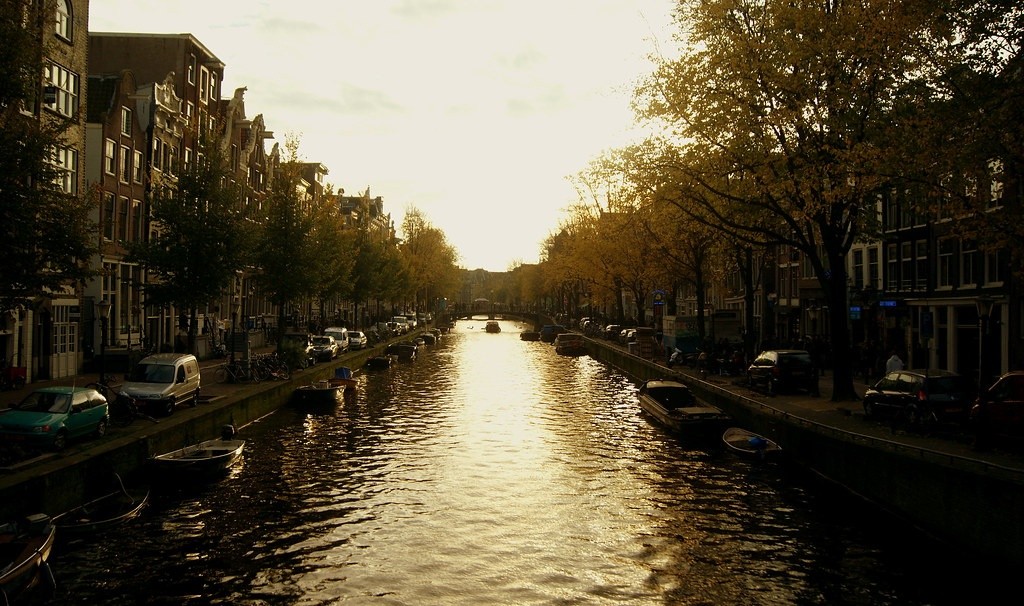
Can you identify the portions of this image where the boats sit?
[486,321,502,333]
[0,514,55,606]
[50,486,150,536]
[151,424,245,473]
[293,366,360,409]
[520,325,584,354]
[637,378,732,435]
[723,428,782,464]
[365,323,455,367]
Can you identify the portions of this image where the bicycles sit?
[213,352,289,383]
[84,375,138,425]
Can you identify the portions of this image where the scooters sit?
[667,341,741,378]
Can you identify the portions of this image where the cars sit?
[579,317,637,343]
[285,311,435,365]
[0,387,110,452]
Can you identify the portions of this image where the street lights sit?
[337,302,342,325]
[491,290,493,300]
[972,291,996,393]
[806,304,821,371]
[230,301,240,365]
[96,298,113,347]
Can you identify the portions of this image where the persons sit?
[886,350,903,385]
[735,329,833,375]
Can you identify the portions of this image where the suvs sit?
[748,349,815,397]
[971,371,1024,441]
[119,353,201,417]
[863,369,968,439]
